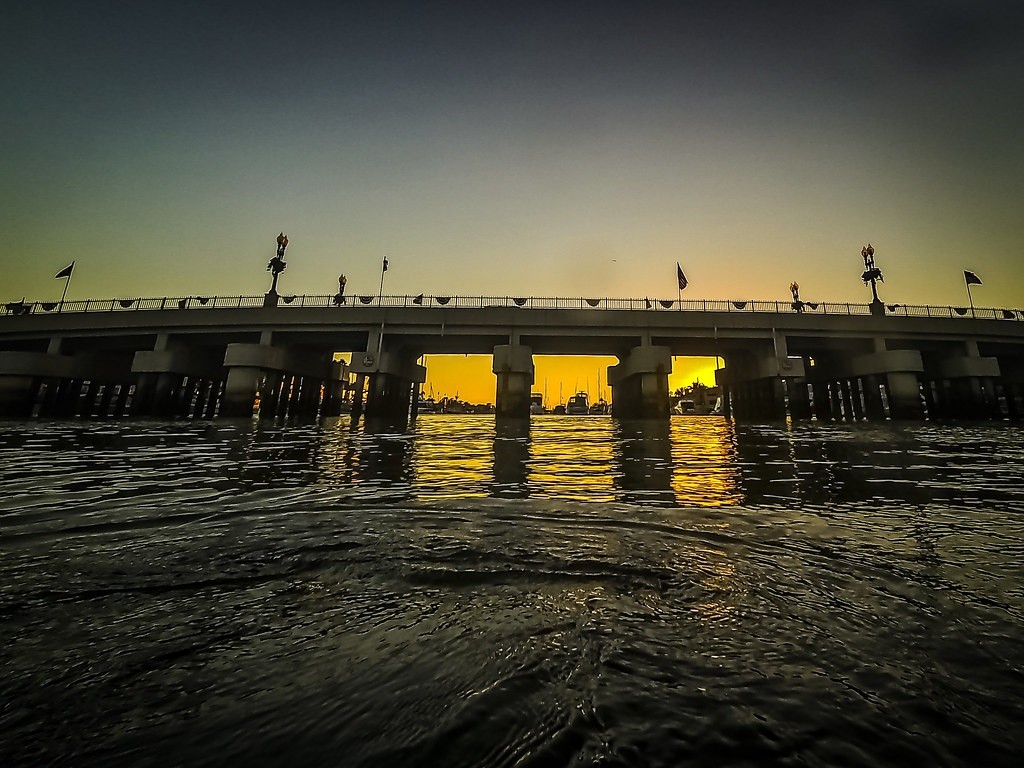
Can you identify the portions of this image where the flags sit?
[963,270,984,285]
[54,264,73,277]
[679,269,688,291]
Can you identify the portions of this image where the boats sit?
[335,389,734,418]
